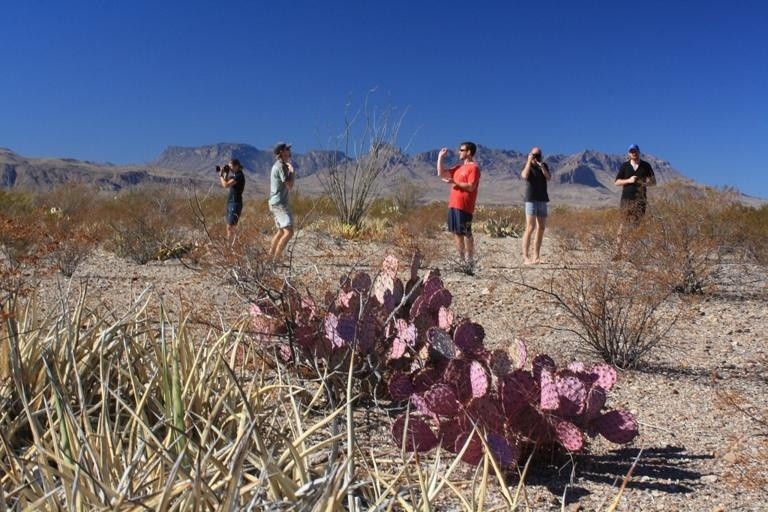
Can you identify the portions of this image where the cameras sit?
[216,165,229,174]
[533,153,542,162]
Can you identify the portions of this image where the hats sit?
[272,141,293,156]
[626,143,640,153]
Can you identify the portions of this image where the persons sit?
[216,158,245,242]
[609,142,657,262]
[266,142,297,269]
[436,141,481,269]
[519,147,551,266]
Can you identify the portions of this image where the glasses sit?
[459,148,470,152]
